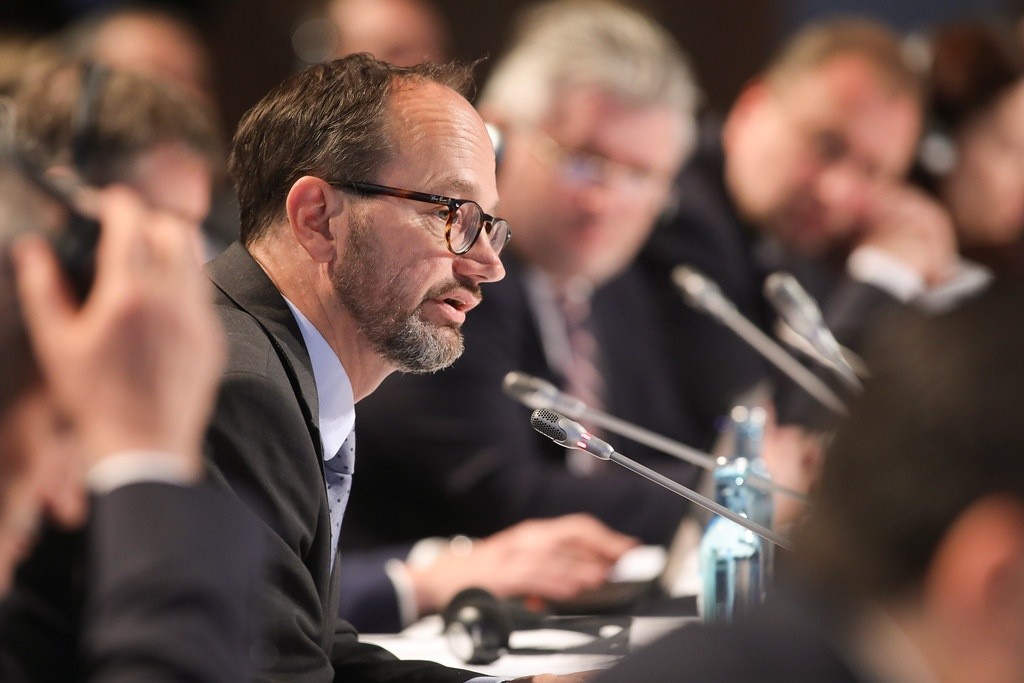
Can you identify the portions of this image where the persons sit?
[1,0,1024,683]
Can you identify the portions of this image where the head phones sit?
[440,587,634,665]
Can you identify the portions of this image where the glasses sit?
[508,122,671,205]
[286,181,512,256]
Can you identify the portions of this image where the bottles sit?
[697,464,765,624]
[733,420,776,531]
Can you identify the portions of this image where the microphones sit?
[500,262,872,550]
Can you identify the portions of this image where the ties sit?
[556,278,600,474]
[318,430,353,576]
